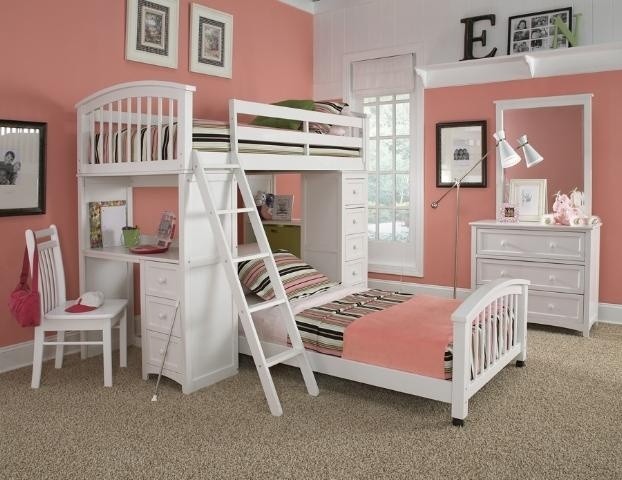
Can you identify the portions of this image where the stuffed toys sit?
[552,191,573,226]
[570,187,583,214]
[254,191,273,221]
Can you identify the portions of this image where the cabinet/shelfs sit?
[300,170,370,292]
[140,259,242,395]
[468,218,603,337]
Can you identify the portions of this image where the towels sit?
[342,292,508,380]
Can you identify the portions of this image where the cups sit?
[121,226,141,249]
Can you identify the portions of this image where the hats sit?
[65,290,105,314]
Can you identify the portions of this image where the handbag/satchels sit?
[7,282,41,328]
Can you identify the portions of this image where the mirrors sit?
[492,94,593,223]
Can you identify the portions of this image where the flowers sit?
[567,186,584,213]
[552,190,577,225]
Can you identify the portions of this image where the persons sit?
[0,151,21,185]
[513,14,566,53]
[454,149,469,160]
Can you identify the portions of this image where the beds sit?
[72,81,370,394]
[226,241,531,425]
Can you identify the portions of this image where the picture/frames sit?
[0,118,48,217]
[272,194,294,221]
[125,0,180,69]
[507,7,572,56]
[189,2,233,79]
[509,178,546,221]
[435,119,486,188]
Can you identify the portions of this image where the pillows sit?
[250,98,315,130]
[234,248,342,304]
[301,98,349,134]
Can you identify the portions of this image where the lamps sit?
[430,130,523,299]
[512,134,544,170]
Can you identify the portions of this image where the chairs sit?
[25,224,130,388]
